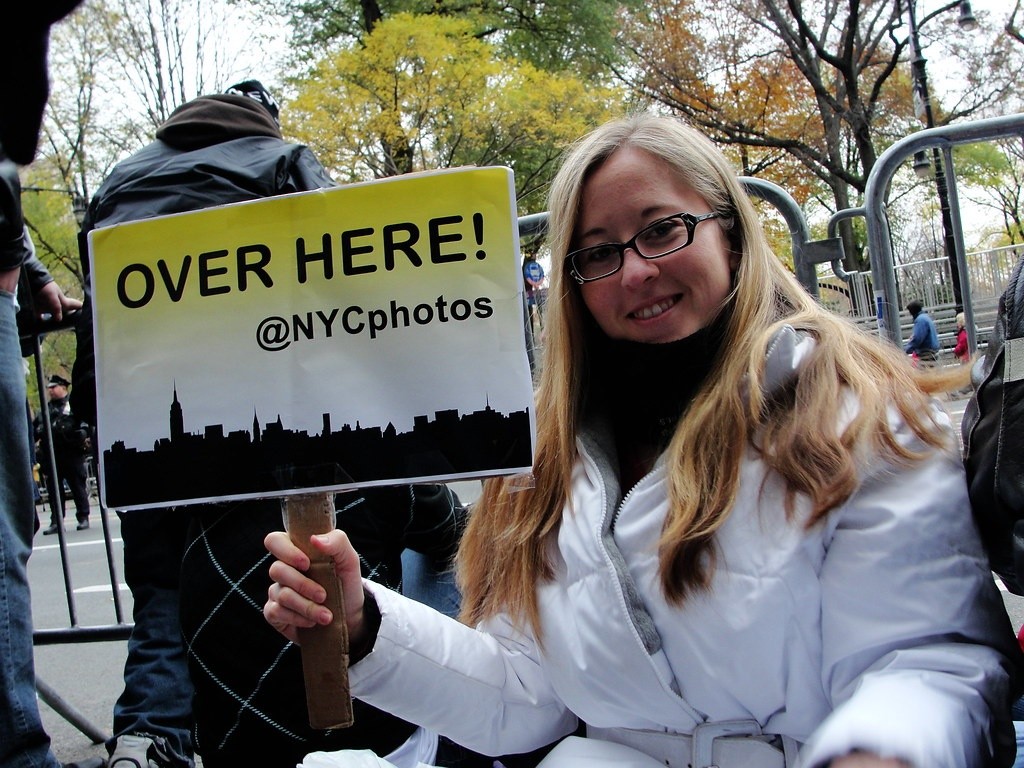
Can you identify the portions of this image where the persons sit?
[904,300,959,402]
[264,116,1024,768]
[954,312,973,397]
[0,0,107,768]
[31,375,90,535]
[69,79,472,768]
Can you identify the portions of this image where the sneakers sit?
[102,731,184,768]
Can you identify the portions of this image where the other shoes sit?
[41,524,57,537]
[74,518,92,532]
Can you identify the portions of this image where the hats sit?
[222,76,281,125]
[906,301,922,315]
[43,374,72,389]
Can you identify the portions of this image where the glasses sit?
[562,208,732,285]
